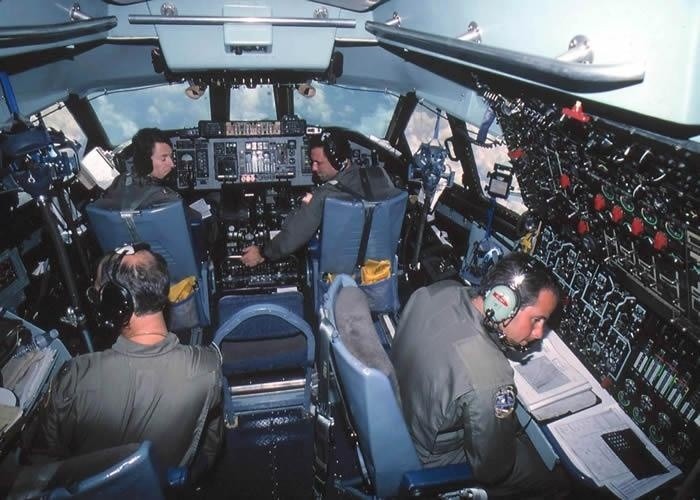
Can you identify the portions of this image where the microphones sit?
[482,318,540,353]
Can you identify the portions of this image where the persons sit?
[238,127,396,270]
[99,126,192,223]
[20,239,225,482]
[387,251,575,500]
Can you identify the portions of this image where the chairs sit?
[84,198,216,347]
[207,291,320,447]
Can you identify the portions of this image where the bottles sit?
[11,328,60,358]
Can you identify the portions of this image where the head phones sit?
[133,128,160,177]
[483,259,539,323]
[320,130,348,172]
[92,241,151,338]
